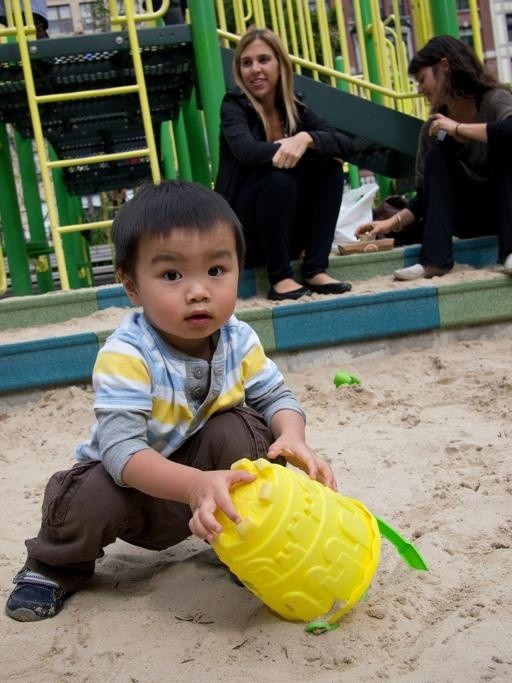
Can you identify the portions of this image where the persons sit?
[2,178,340,623]
[212,27,355,301]
[352,34,512,281]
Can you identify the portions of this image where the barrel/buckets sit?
[209,458,428,634]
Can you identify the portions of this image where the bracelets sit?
[455,122,463,136]
[393,214,402,232]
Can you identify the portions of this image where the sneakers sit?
[302,279,353,295]
[4,567,77,622]
[267,284,314,302]
[393,262,447,281]
